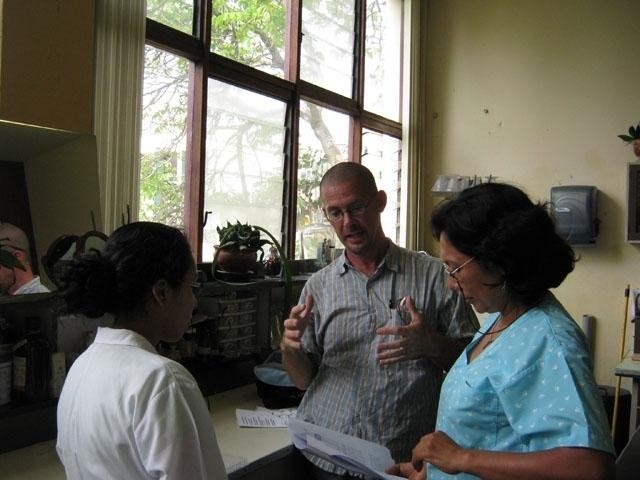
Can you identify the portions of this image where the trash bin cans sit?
[597,385,631,458]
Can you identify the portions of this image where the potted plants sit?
[617,123,640,156]
[213,220,272,273]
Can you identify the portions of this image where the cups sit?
[431,174,495,192]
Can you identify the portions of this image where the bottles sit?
[264,247,282,277]
[0,312,53,406]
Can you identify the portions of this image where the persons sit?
[0,223,51,295]
[386,181,617,480]
[54,221,229,480]
[279,162,481,480]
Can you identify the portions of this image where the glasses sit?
[327,194,378,223]
[442,255,478,281]
